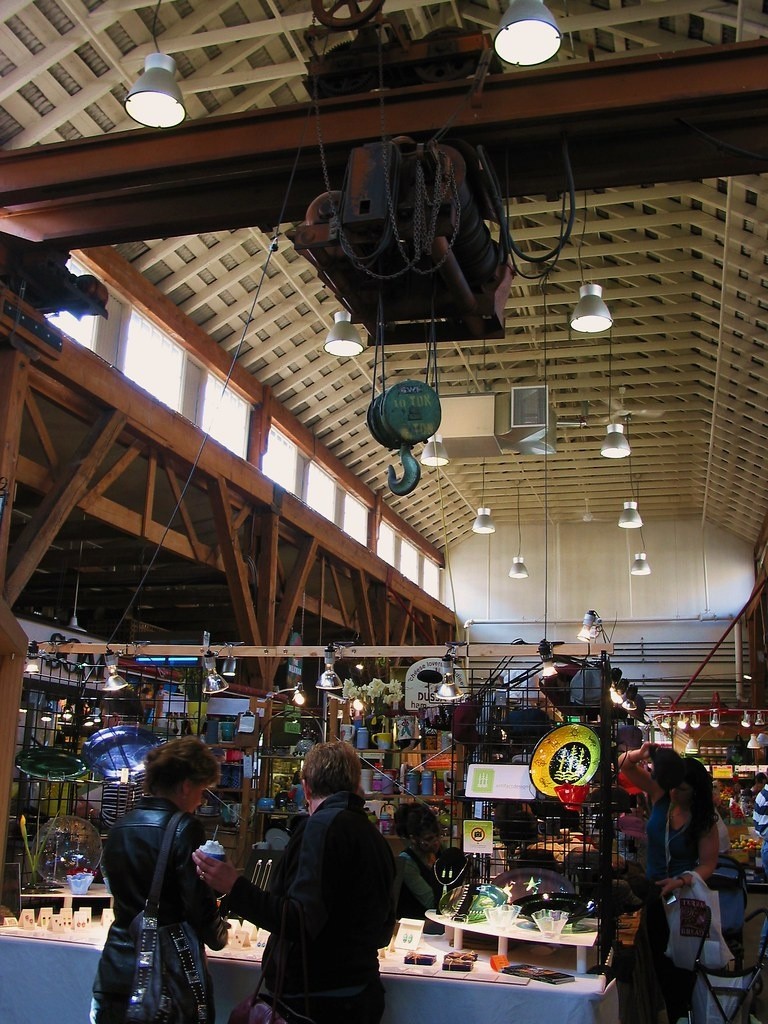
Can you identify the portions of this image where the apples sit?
[731,839,762,849]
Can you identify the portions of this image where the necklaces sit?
[667,810,681,821]
[410,846,436,868]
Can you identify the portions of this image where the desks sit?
[20,883,114,915]
[0,909,606,1024]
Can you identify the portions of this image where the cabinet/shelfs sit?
[685,738,755,780]
[140,694,464,878]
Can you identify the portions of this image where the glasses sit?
[415,832,447,845]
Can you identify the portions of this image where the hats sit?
[612,879,642,909]
[452,703,479,745]
[647,744,683,790]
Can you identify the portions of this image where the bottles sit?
[356,727,451,833]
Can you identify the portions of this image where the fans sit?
[566,498,614,523]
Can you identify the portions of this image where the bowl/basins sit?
[395,739,421,751]
[201,806,214,814]
[510,892,598,926]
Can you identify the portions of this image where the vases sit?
[365,715,382,749]
[188,702,208,735]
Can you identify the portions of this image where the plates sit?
[439,884,508,922]
[198,813,220,816]
[265,828,290,850]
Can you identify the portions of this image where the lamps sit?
[222,656,236,676]
[124,0,186,129]
[421,435,449,467]
[83,699,101,726]
[747,734,760,749]
[67,513,88,632]
[508,485,529,578]
[103,649,128,690]
[741,714,750,728]
[568,190,613,332]
[471,457,495,533]
[661,715,671,728]
[62,705,72,718]
[619,416,644,528]
[315,643,343,689]
[755,713,765,726]
[631,479,650,575]
[324,311,365,356]
[538,639,558,677]
[576,610,596,642]
[41,702,52,722]
[609,667,637,711]
[600,328,633,459]
[436,654,464,699]
[709,711,720,727]
[202,650,229,693]
[678,713,689,730]
[688,714,701,728]
[685,738,698,754]
[494,0,562,66]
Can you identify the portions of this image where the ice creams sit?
[198,839,225,854]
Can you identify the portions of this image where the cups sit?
[199,850,226,863]
[251,842,271,850]
[372,733,392,750]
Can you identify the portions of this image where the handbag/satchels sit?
[129,922,221,1023]
[664,874,736,969]
[227,991,322,1024]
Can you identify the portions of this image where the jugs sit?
[227,804,242,822]
[339,724,355,746]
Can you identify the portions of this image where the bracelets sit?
[677,877,686,888]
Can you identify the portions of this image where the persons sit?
[89,735,231,1024]
[140,684,164,727]
[168,712,178,737]
[192,741,397,1024]
[181,715,191,738]
[101,712,120,730]
[389,802,448,921]
[619,741,768,1024]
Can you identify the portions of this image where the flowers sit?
[173,667,210,702]
[341,678,405,726]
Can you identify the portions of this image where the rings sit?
[200,871,207,881]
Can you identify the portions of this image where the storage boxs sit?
[405,954,436,966]
[442,962,473,971]
[444,953,478,962]
[727,850,749,862]
